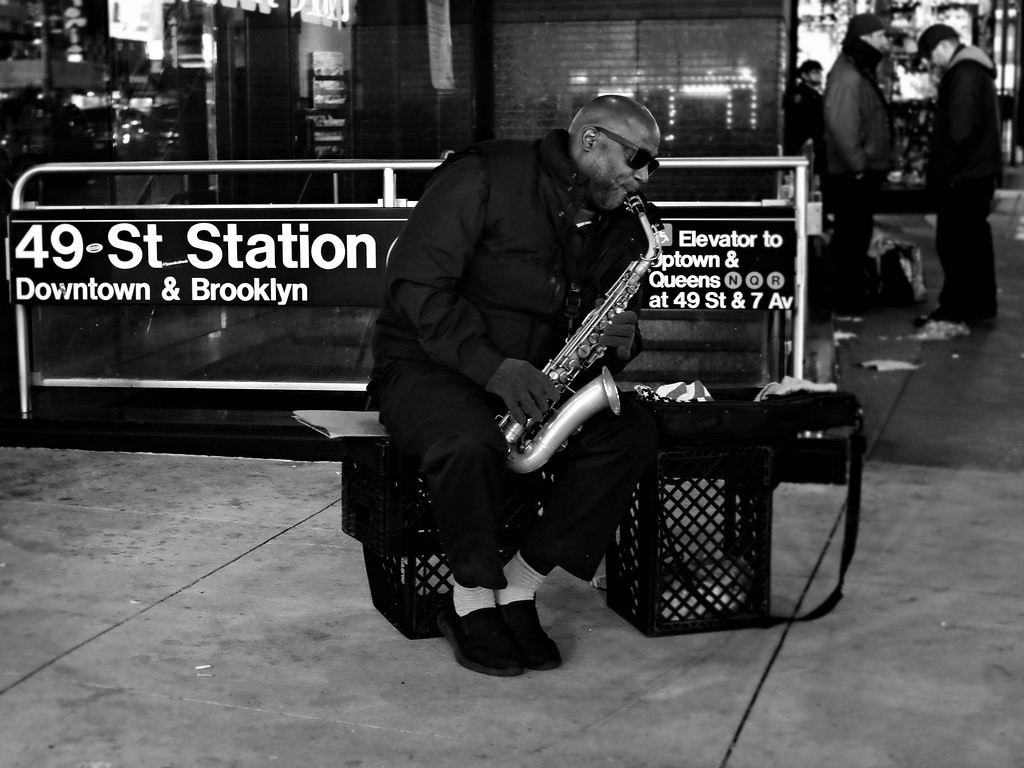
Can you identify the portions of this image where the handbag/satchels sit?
[867,233,929,307]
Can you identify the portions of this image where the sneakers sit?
[437,599,562,676]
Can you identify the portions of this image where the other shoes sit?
[914,315,994,329]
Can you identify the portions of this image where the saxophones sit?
[497,190,686,473]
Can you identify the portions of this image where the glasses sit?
[594,126,660,177]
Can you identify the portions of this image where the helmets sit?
[797,60,823,77]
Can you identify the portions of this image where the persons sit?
[815,12,889,320]
[367,95,663,676]
[910,24,1000,327]
[782,61,833,234]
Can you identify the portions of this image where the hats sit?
[847,13,885,37]
[912,24,960,67]
